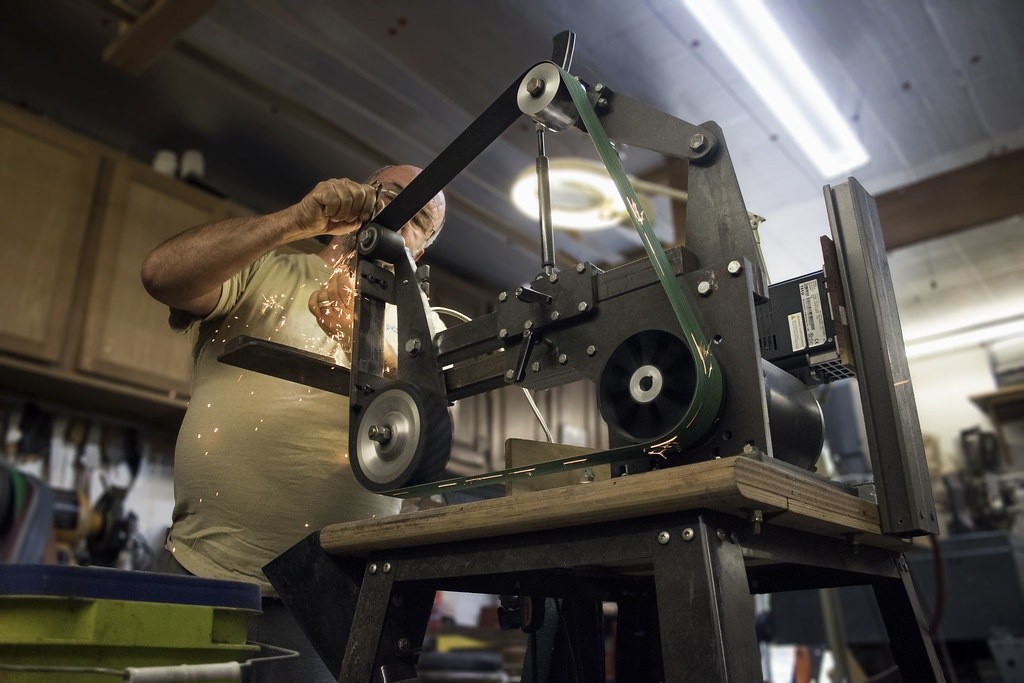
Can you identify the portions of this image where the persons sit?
[140,165,449,683]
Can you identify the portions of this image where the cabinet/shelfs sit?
[0,109,608,479]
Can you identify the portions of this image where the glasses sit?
[375,189,436,242]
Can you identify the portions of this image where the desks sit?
[319,456,944,683]
